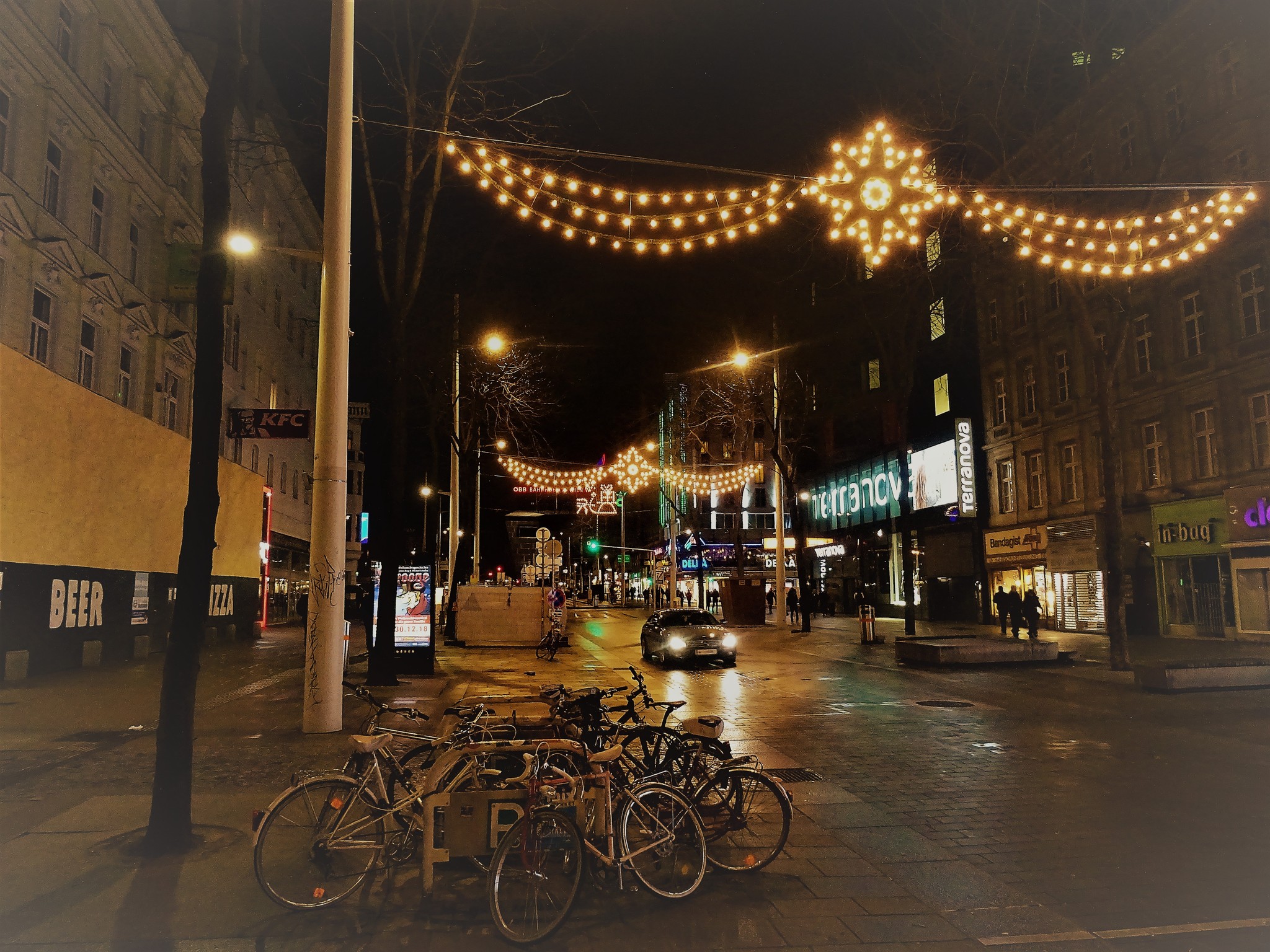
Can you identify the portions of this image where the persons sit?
[854,588,864,617]
[812,589,818,619]
[993,586,1009,634]
[706,589,712,611]
[786,588,799,624]
[1023,590,1043,638]
[819,588,827,617]
[625,586,692,609]
[766,590,776,614]
[711,589,720,613]
[827,587,838,615]
[1007,585,1023,637]
[296,594,308,654]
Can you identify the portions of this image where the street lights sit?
[733,320,791,627]
[420,472,433,553]
[645,442,678,611]
[451,331,505,625]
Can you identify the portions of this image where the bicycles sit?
[249,668,796,944]
[532,616,565,661]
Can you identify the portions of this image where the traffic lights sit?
[497,567,501,572]
[515,579,520,583]
[488,572,493,577]
[589,542,599,551]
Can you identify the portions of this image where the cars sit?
[640,606,738,664]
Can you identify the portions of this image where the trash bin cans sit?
[857,604,876,643]
